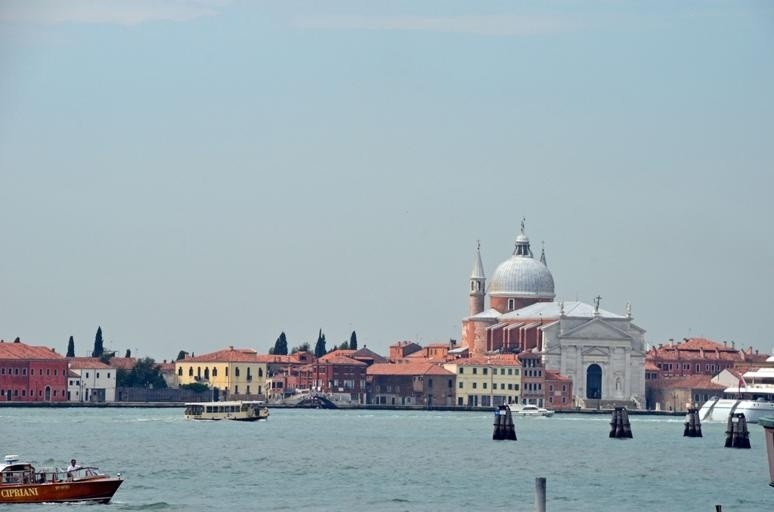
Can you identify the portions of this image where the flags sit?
[739,375,744,384]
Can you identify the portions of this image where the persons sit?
[66,458,82,482]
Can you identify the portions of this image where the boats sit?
[518,404,555,417]
[0,454,125,505]
[183,400,270,420]
[698,356,774,425]
